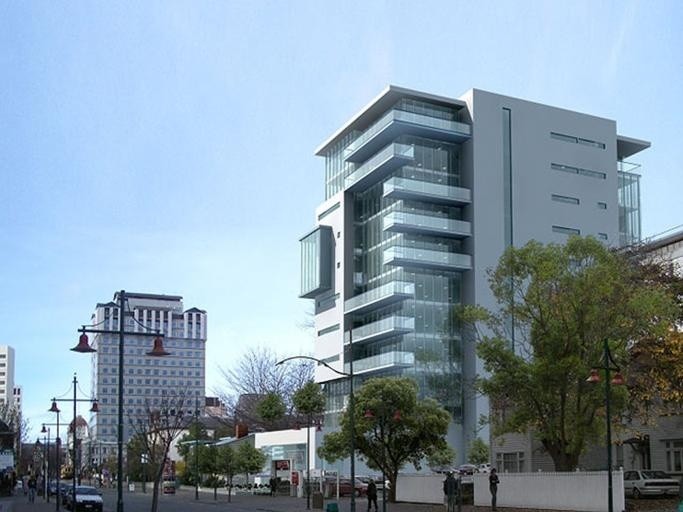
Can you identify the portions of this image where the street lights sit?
[275,316,356,512]
[37,372,100,512]
[364,400,400,512]
[70,290,171,512]
[586,337,626,512]
[296,415,321,510]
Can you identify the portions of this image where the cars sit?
[431,463,491,475]
[326,476,383,497]
[624,469,680,500]
[50,482,103,512]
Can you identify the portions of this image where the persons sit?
[22,477,29,498]
[443,471,457,511]
[27,475,37,504]
[365,478,378,512]
[488,467,499,511]
[269,474,277,496]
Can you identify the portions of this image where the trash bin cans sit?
[312,492,324,509]
